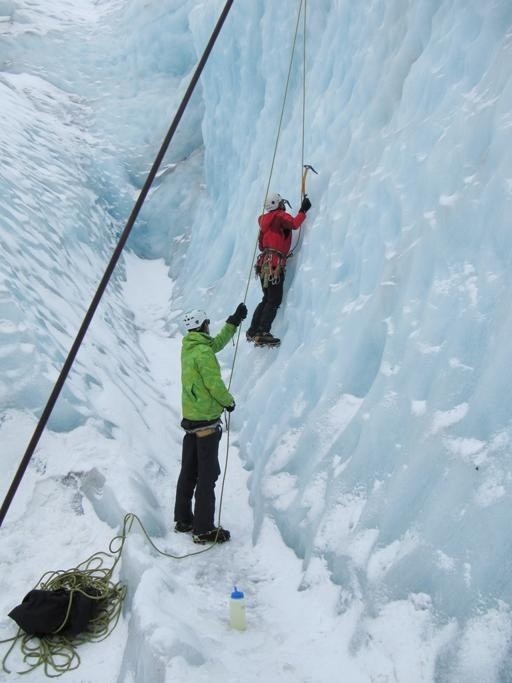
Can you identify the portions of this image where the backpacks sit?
[7,588,91,633]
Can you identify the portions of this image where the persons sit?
[246,195,314,347]
[174,303,244,544]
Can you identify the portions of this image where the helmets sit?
[184,308,210,329]
[264,191,282,212]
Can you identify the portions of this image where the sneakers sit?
[246,331,281,350]
[174,521,230,545]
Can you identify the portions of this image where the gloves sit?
[227,304,247,327]
[299,198,312,214]
[227,401,236,413]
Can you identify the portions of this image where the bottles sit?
[228,584,247,631]
